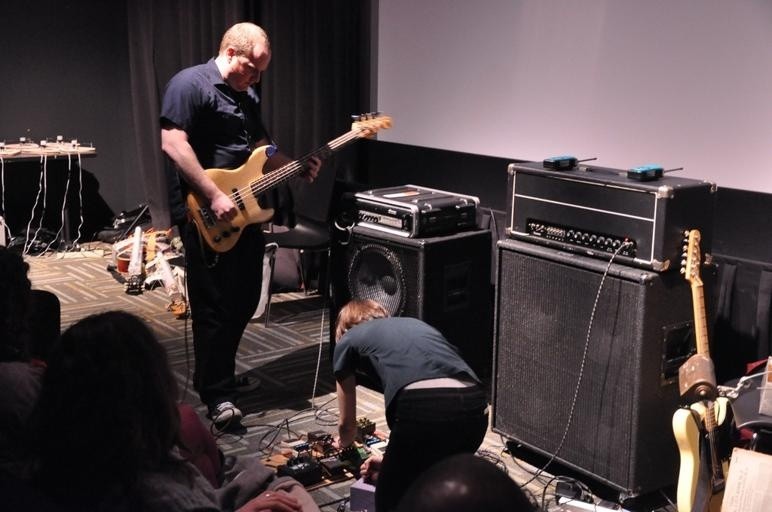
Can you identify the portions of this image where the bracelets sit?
[337,437,355,451]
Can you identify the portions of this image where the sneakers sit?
[212,399,243,426]
[218,375,261,393]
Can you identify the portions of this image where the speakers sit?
[331,226,492,395]
[491,240,718,496]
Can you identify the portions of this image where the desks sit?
[0,142,98,242]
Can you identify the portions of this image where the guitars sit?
[671,230,737,512]
[187,112,393,252]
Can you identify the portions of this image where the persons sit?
[332,297,489,510]
[155,23,321,428]
[1,243,237,491]
[393,453,539,511]
[36,314,324,512]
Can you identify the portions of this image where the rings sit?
[266,493,271,497]
